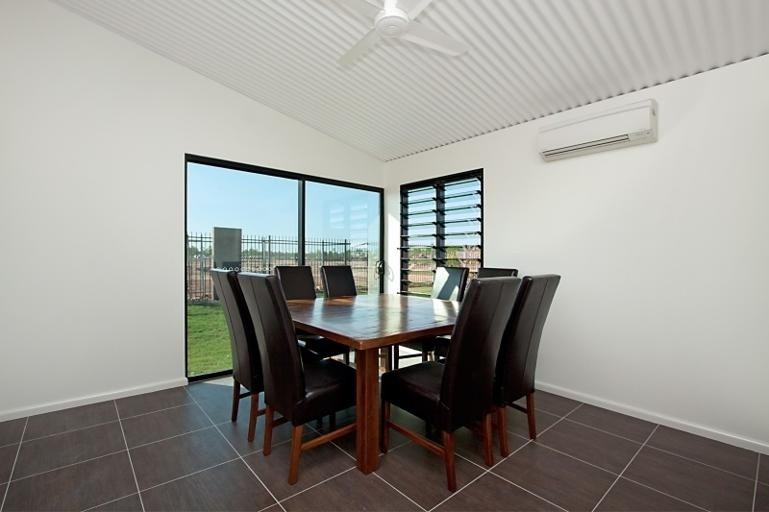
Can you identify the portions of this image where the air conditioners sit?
[539,98,659,163]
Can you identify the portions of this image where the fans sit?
[336,0,469,67]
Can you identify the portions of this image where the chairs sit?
[392,266,467,370]
[427,273,560,458]
[210,268,337,455]
[321,266,358,361]
[379,276,522,491]
[434,267,519,360]
[275,265,349,365]
[238,272,360,485]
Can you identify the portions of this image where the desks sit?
[283,295,461,475]
